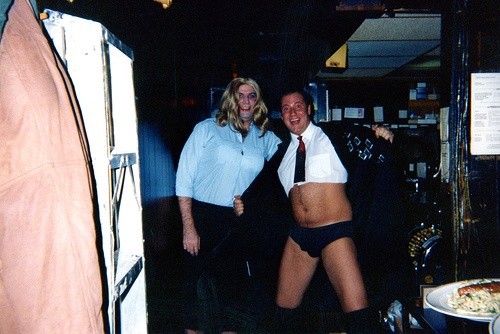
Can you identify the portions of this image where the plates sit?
[489,313,500,334]
[425,278,500,323]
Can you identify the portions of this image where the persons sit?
[175,78,284,333]
[233,87,394,334]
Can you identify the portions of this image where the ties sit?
[293,136,306,183]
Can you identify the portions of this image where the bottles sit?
[406,164,420,204]
